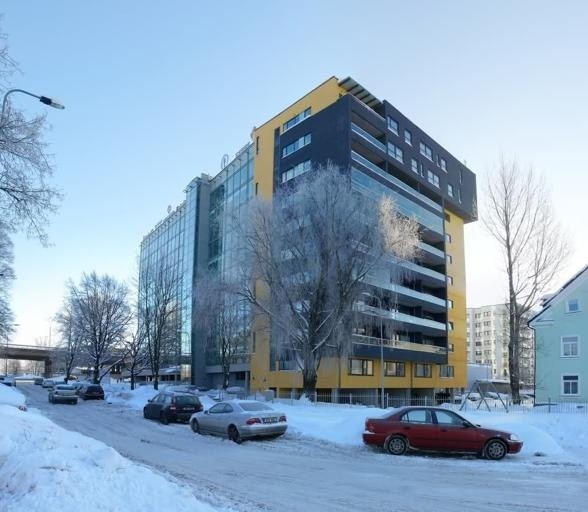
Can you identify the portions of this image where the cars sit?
[0,376,16,387]
[362,406,524,460]
[143,391,289,444]
[34,377,104,405]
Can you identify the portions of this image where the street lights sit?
[5,324,21,376]
[0,89,66,163]
